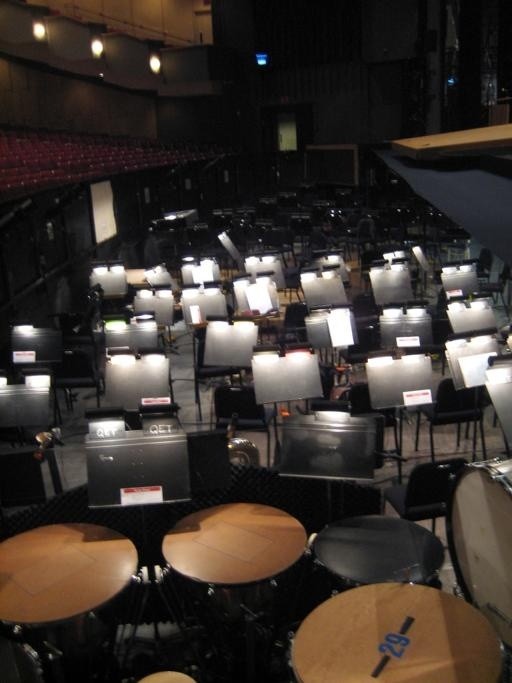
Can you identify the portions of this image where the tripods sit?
[161,325,181,355]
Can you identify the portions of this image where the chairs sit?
[384,458,469,537]
[471,248,510,317]
[191,326,244,421]
[49,336,104,413]
[132,198,432,280]
[209,377,490,462]
[279,302,313,348]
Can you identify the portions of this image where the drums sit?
[445,457,511,648]
[289,579,506,683]
[162,503,309,618]
[1,522,141,644]
[311,515,445,602]
